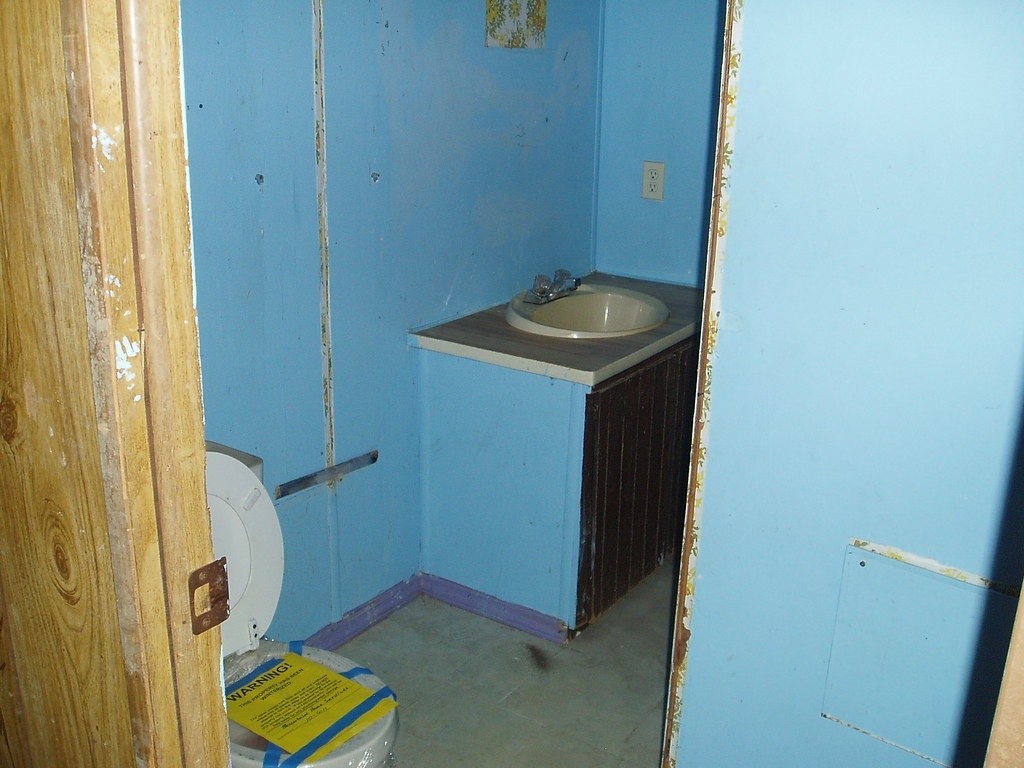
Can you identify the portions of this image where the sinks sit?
[504,280,671,340]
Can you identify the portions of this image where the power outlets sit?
[642,162,663,200]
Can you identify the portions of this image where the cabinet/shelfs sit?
[574,336,700,628]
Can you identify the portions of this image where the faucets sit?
[539,272,583,304]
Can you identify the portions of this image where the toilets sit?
[205,435,401,766]
